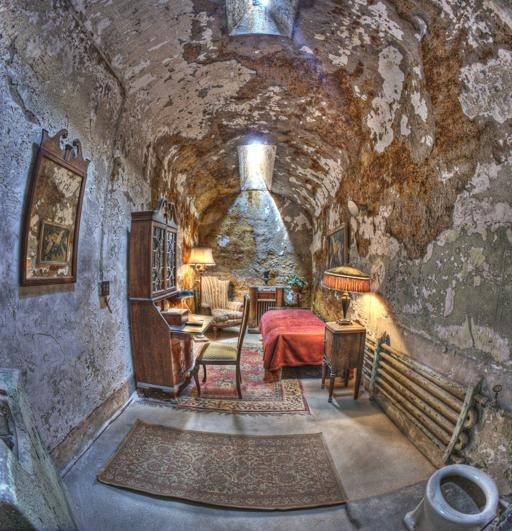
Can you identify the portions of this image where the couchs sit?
[193,277,248,337]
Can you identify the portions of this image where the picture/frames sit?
[17,127,92,287]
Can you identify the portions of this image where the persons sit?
[45,229,66,260]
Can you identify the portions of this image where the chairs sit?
[192,294,251,401]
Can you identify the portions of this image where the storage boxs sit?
[160,306,190,325]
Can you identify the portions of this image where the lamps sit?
[183,247,216,314]
[320,263,371,327]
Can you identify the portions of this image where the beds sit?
[259,308,326,384]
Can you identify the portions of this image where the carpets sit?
[93,418,350,514]
[135,341,313,417]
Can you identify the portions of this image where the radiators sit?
[352,316,488,468]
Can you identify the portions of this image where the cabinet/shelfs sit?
[127,198,215,400]
[321,321,366,404]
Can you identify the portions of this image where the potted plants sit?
[284,271,307,291]
